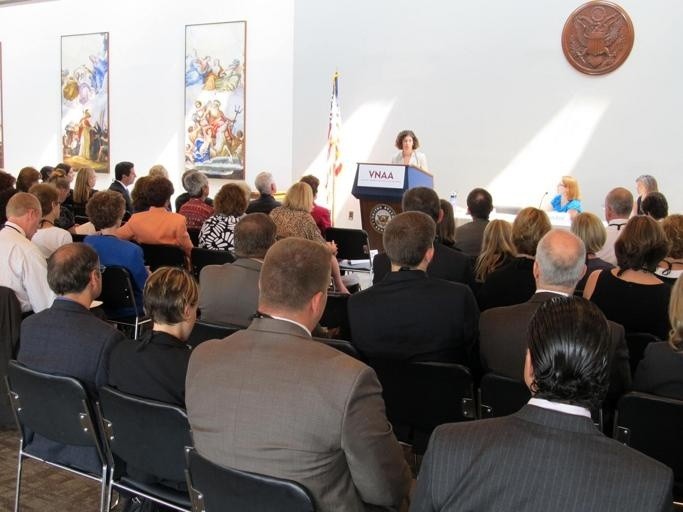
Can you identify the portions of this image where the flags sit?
[322,78,347,188]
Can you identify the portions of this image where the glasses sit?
[98,265,106,273]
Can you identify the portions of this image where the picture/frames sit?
[183,19,244,182]
[61,32,108,174]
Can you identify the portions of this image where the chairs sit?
[478,375,607,437]
[312,334,357,356]
[0,287,20,362]
[94,386,193,511]
[324,291,353,334]
[179,444,317,512]
[139,243,189,273]
[70,233,86,243]
[370,362,474,461]
[98,266,153,341]
[610,392,683,511]
[75,216,89,224]
[190,247,232,284]
[190,320,245,351]
[3,363,109,511]
[321,227,373,272]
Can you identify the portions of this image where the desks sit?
[353,163,431,264]
[450,208,594,229]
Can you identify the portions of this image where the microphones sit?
[536,192,548,209]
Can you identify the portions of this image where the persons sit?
[198,183,247,258]
[583,216,671,340]
[15,167,41,191]
[638,193,668,219]
[185,236,413,511]
[484,208,551,310]
[45,172,75,228]
[84,191,151,320]
[298,175,331,230]
[174,170,213,212]
[42,162,71,182]
[0,193,105,319]
[479,228,632,396]
[70,166,98,215]
[391,130,427,171]
[373,186,473,284]
[109,162,136,213]
[476,219,514,278]
[0,170,16,198]
[408,296,675,511]
[660,215,683,269]
[268,182,351,294]
[148,165,167,178]
[28,184,72,258]
[633,270,683,400]
[435,200,455,244]
[18,244,129,477]
[246,172,281,212]
[456,189,494,255]
[546,176,582,220]
[634,175,659,194]
[199,213,339,338]
[569,212,613,270]
[179,172,214,227]
[107,267,199,497]
[348,210,479,369]
[599,187,635,267]
[115,176,195,252]
